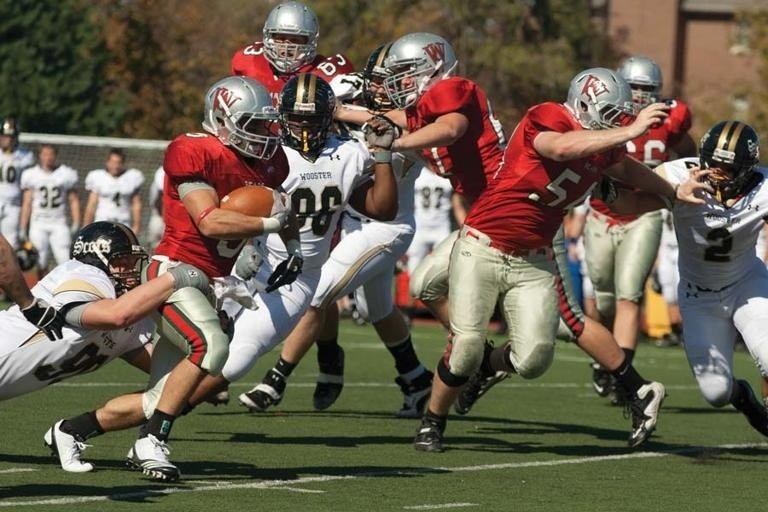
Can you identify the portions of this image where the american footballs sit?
[219,185,287,218]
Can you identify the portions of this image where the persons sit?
[45,2,768,482]
[0,231,74,342]
[0,219,236,405]
[16,142,83,282]
[1,109,36,304]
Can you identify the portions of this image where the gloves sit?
[361,115,394,163]
[166,262,208,296]
[265,254,302,293]
[234,245,262,282]
[20,297,70,341]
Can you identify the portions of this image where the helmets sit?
[69,220,150,298]
[361,41,399,113]
[382,31,459,112]
[201,74,282,163]
[563,66,634,130]
[261,0,319,75]
[698,120,759,202]
[278,73,336,152]
[0,117,21,134]
[616,56,662,112]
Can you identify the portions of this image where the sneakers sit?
[627,380,666,450]
[42,417,97,474]
[454,340,496,415]
[123,432,180,483]
[732,379,767,436]
[237,366,287,412]
[393,364,436,420]
[311,341,345,410]
[414,408,447,454]
[591,358,612,398]
